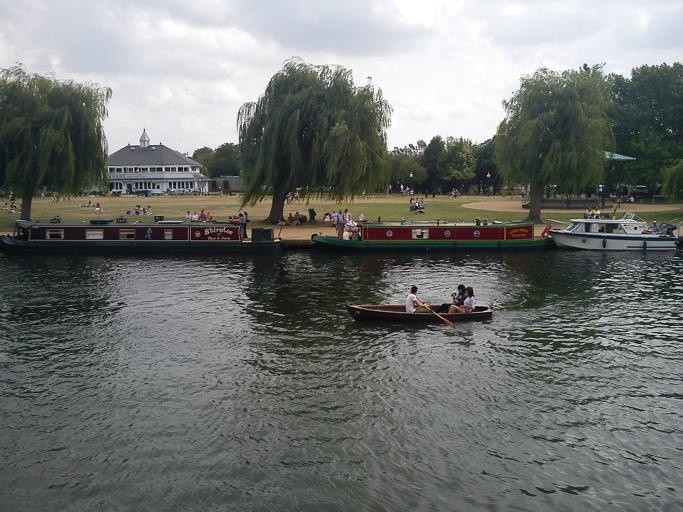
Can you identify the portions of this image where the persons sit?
[107,189,112,198]
[287,213,297,226]
[95,203,100,211]
[182,208,213,224]
[407,196,426,215]
[225,211,249,224]
[88,200,92,207]
[439,283,467,313]
[583,208,593,220]
[294,211,301,225]
[404,285,431,313]
[644,220,660,236]
[48,215,62,225]
[592,207,600,219]
[320,208,360,242]
[450,187,461,199]
[119,202,152,218]
[446,286,475,313]
[0,202,8,210]
[7,201,16,213]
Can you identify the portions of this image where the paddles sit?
[416,297,455,329]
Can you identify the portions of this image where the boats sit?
[548,212,680,251]
[345,303,492,323]
[310,221,549,250]
[1,219,281,250]
